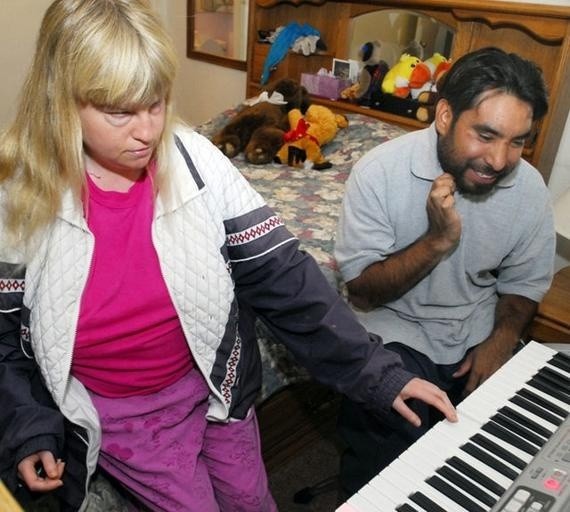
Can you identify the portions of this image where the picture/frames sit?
[185,0,251,72]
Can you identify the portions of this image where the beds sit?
[1,97,413,512]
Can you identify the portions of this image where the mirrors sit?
[344,8,458,72]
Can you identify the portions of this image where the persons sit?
[328,45,557,503]
[0,1,461,511]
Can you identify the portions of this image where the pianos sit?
[334,340,570,512]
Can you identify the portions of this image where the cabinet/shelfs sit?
[246,42,336,100]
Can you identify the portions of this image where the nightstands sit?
[521,232,570,345]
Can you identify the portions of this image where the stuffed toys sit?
[415,68,452,123]
[212,78,309,164]
[271,103,349,171]
[380,40,448,112]
[337,40,391,109]
